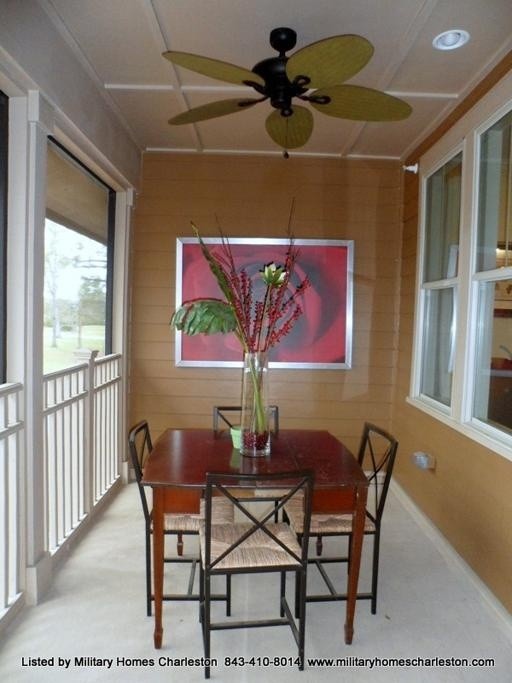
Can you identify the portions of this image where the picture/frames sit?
[172,235,356,369]
[493,240,512,318]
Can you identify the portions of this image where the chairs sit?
[212,404,279,432]
[194,463,318,680]
[281,421,398,618]
[122,419,234,615]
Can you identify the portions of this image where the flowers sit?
[166,237,315,446]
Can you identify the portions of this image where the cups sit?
[229,425,241,449]
[228,449,241,468]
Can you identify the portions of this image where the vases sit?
[239,350,272,457]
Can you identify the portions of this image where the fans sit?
[156,24,414,160]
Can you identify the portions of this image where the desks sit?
[139,426,370,650]
